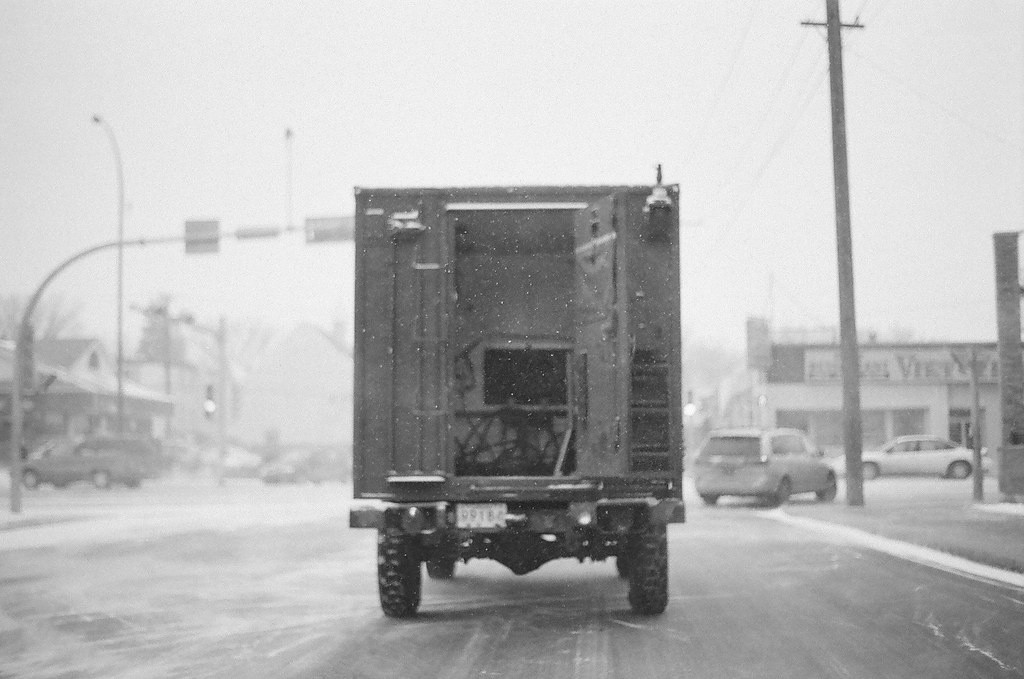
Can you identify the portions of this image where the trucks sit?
[341,169,692,619]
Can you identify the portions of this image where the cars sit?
[255,442,348,484]
[692,426,836,509]
[11,436,146,491]
[836,432,989,477]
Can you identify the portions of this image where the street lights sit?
[90,109,137,438]
